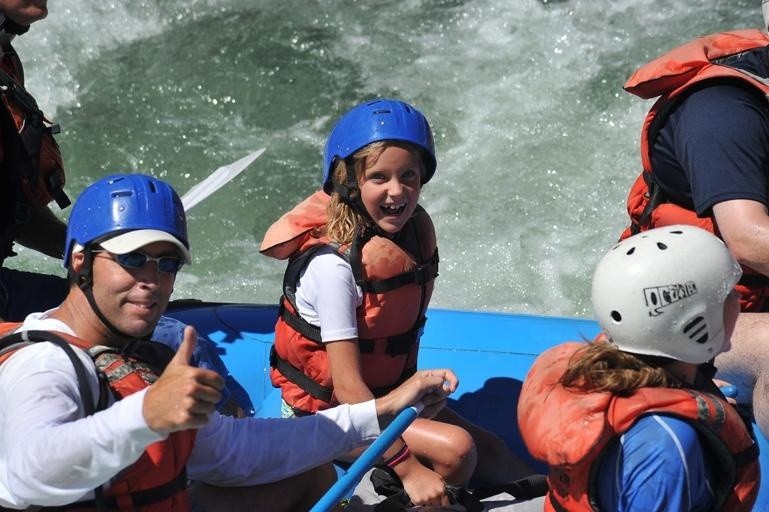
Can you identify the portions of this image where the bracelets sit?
[383,442,406,464]
[386,445,411,470]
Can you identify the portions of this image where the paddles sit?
[179,148,266,212]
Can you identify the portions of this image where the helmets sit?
[63,172,191,268]
[591,224,743,365]
[322,97,437,197]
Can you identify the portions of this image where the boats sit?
[161,299,769,512]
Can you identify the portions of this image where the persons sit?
[0,173,461,509]
[515,221,767,511]
[610,0,768,440]
[256,98,542,512]
[0,1,71,301]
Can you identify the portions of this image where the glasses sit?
[90,250,185,274]
